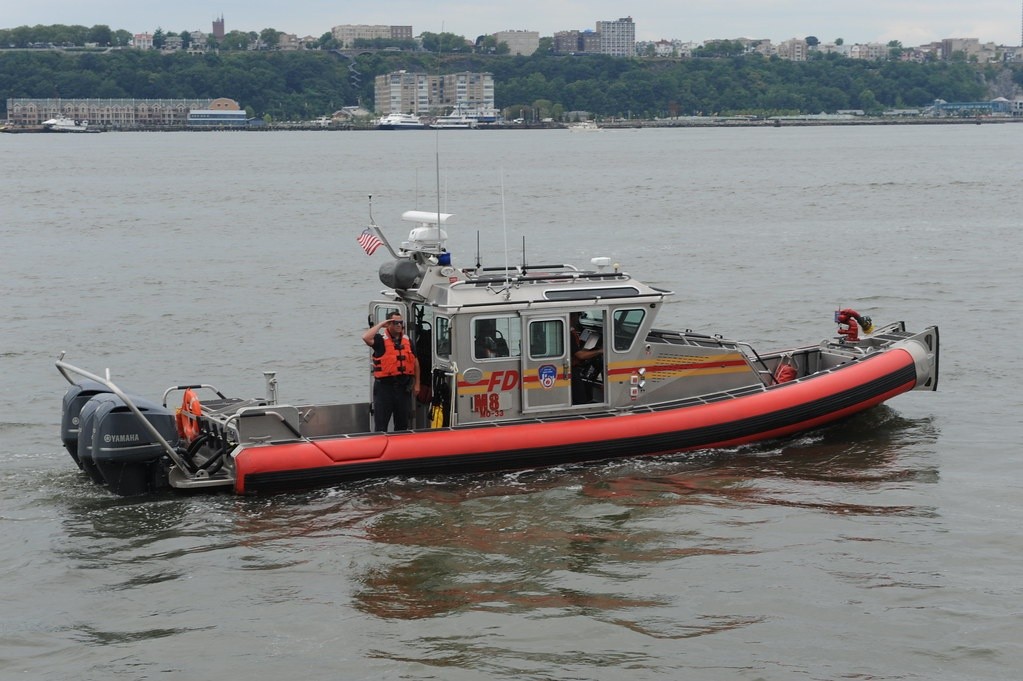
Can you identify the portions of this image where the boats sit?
[40,113,89,133]
[427,102,479,130]
[373,112,426,130]
[51,148,942,500]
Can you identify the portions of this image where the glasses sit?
[390,320,403,325]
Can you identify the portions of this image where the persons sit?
[362,312,420,431]
[569,324,603,404]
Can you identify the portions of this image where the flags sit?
[356,228,385,256]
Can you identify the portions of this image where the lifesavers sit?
[180,389,201,445]
